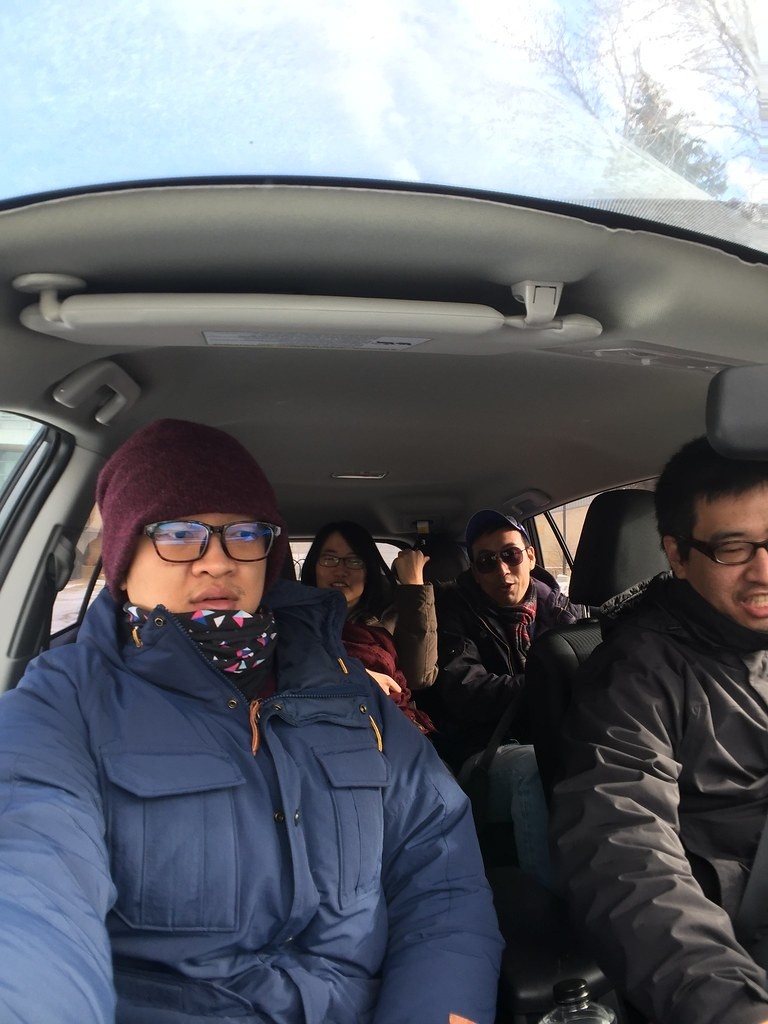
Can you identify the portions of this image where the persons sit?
[441,512,591,783]
[297,523,439,732]
[1,418,505,1024]
[540,435,768,1022]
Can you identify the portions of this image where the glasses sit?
[143,519,281,563]
[675,535,768,566]
[475,547,529,574]
[319,554,364,570]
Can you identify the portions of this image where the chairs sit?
[524,487,672,802]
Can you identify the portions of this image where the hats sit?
[465,509,531,543]
[95,418,288,604]
